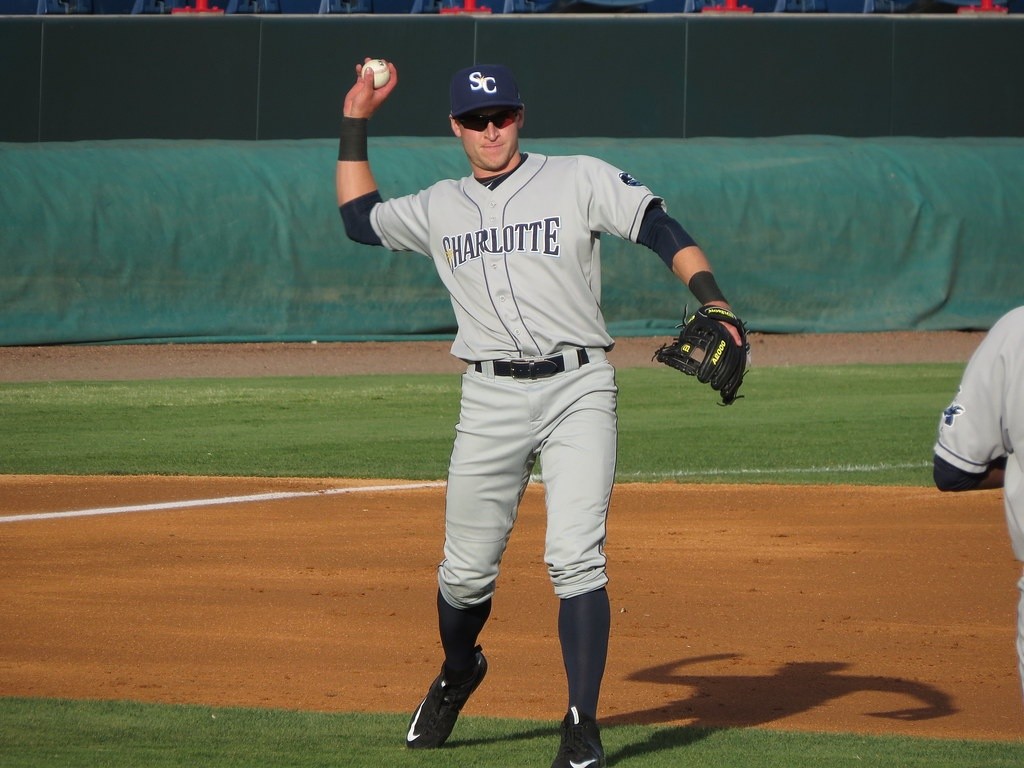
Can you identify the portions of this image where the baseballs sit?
[361,56,390,88]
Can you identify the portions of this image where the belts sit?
[473,347,591,381]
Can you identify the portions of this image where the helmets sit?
[450,65,525,116]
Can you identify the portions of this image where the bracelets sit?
[689,271,729,305]
[338,116,367,161]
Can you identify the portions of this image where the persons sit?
[337,57,750,768]
[930,304,1024,703]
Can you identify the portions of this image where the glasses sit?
[453,106,520,132]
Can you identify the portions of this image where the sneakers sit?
[549,706,605,768]
[406,645,488,750]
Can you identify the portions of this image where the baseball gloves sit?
[658,303,749,406]
